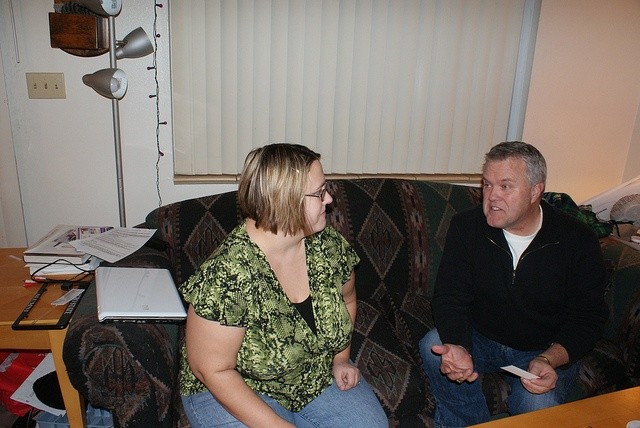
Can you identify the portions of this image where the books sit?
[29,256,101,275]
[22,224,91,264]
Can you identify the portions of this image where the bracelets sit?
[458,344,473,358]
[535,356,551,365]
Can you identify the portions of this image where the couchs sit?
[62,179,640,428]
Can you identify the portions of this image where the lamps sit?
[79,0,154,227]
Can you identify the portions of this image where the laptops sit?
[94,265,187,324]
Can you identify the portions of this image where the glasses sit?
[305,189,326,201]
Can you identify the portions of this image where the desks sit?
[0,247,85,427]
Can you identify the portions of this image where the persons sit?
[176,143,390,427]
[418,141,610,427]
